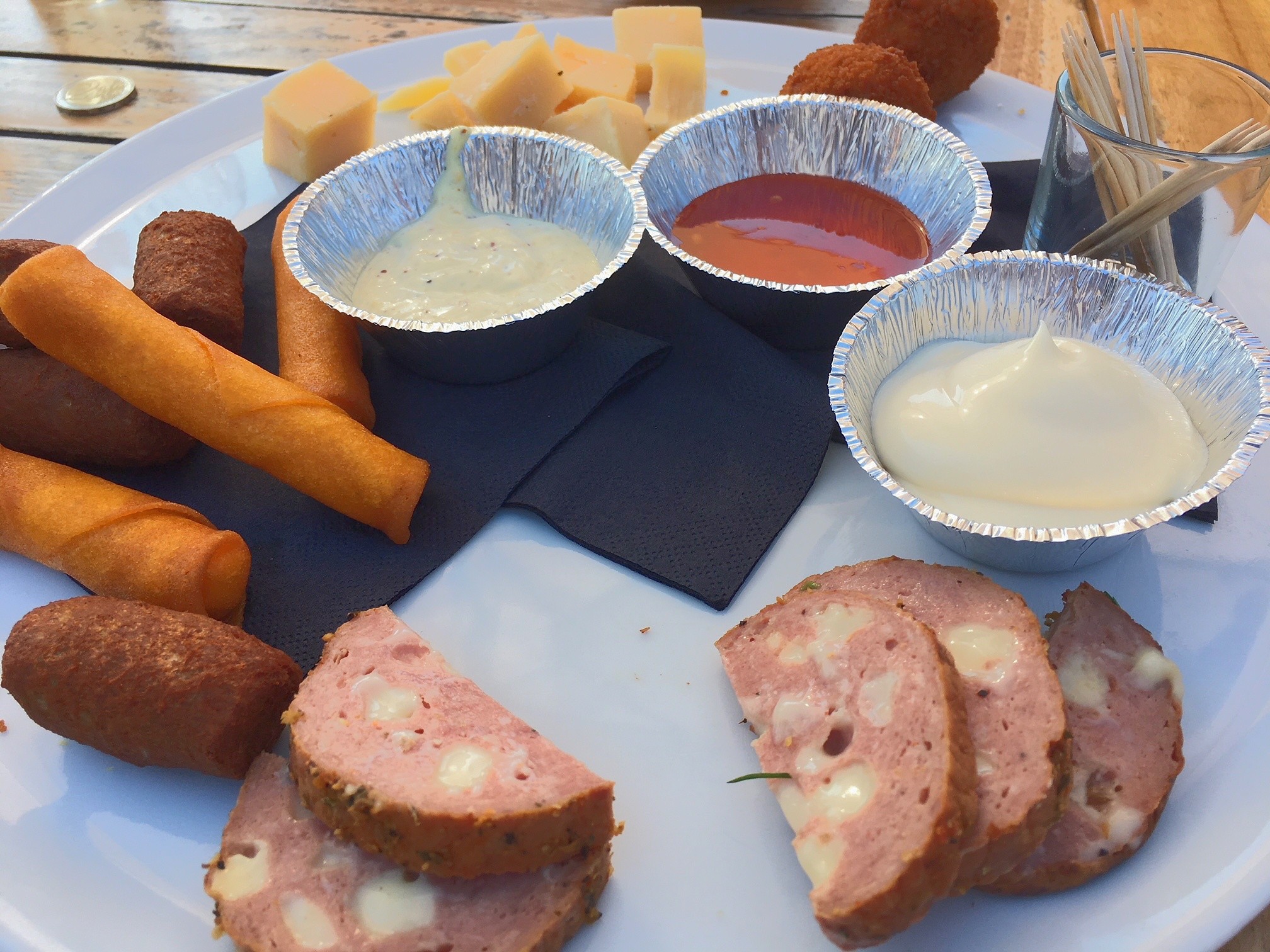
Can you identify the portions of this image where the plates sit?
[0,15,1270,952]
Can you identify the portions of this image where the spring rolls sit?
[0,243,432,546]
[0,444,250,626]
[270,194,376,433]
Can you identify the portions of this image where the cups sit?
[1021,45,1269,301]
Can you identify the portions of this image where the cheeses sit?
[263,5,706,179]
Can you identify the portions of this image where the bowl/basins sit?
[281,126,648,380]
[826,249,1270,574]
[630,92,992,334]
